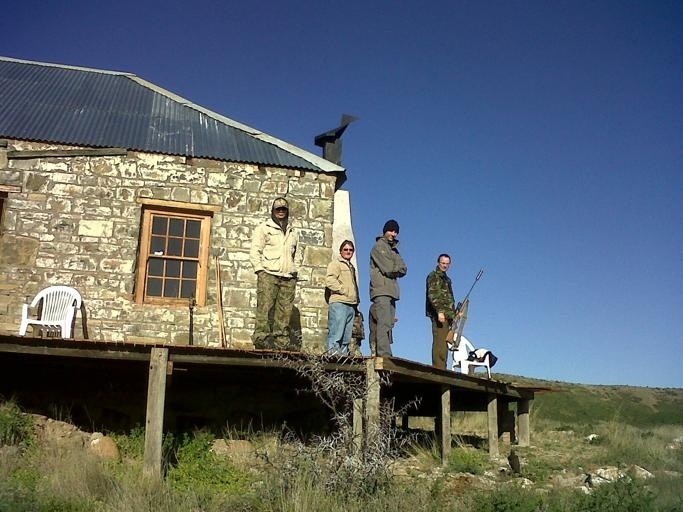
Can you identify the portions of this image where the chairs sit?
[452,333,491,379]
[19,285,82,338]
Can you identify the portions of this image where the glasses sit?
[342,248,354,252]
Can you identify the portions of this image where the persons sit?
[426,253,456,369]
[249,198,299,351]
[368,218,409,357]
[323,239,360,357]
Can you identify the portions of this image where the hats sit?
[273,198,289,210]
[383,219,399,234]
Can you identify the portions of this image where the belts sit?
[273,274,296,282]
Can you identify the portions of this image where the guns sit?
[446,267,484,344]
